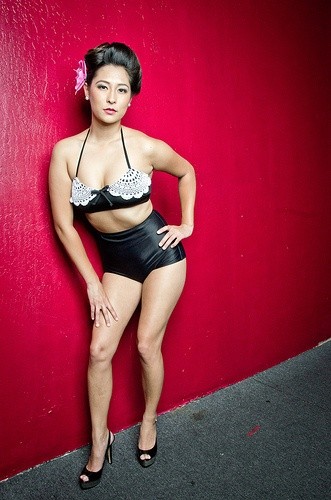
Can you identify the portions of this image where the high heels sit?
[136,436,157,467]
[79,427,115,489]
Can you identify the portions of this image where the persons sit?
[48,41,196,488]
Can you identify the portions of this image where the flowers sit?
[73,58,88,95]
